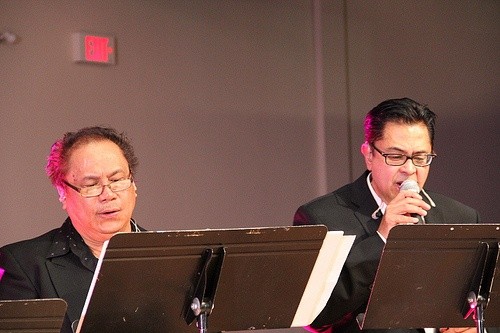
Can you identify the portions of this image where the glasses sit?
[59,173,137,196]
[370,142,440,167]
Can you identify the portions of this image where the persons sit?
[293,97,481,333]
[0,127,148,333]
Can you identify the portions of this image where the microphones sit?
[400,180,425,224]
